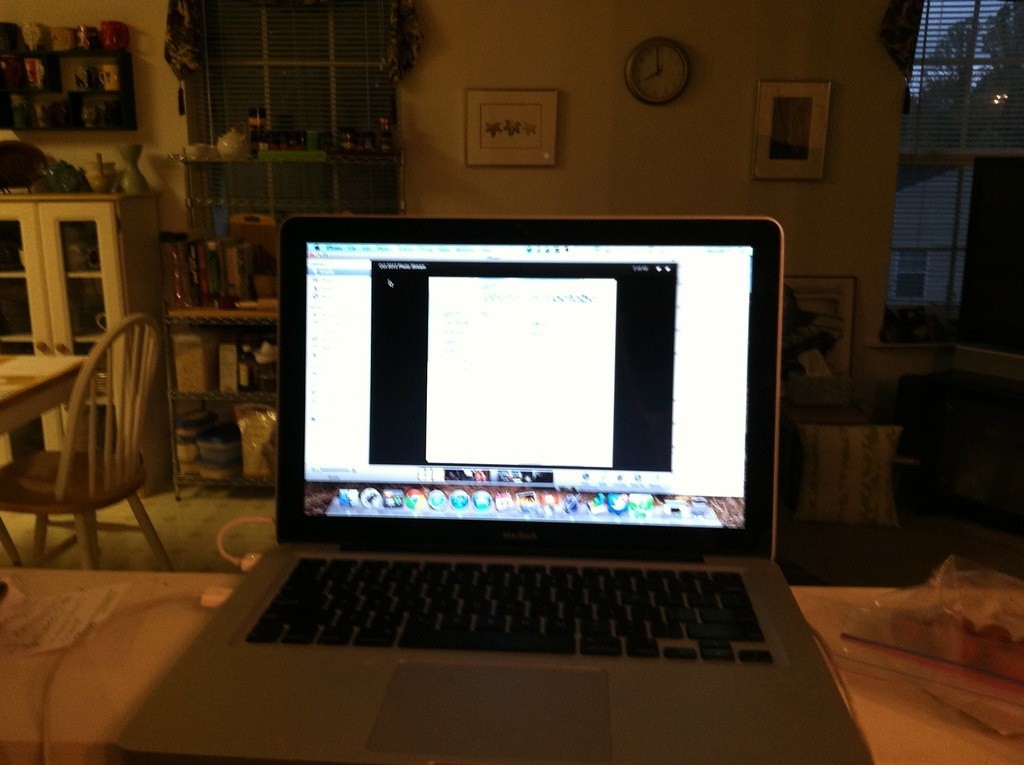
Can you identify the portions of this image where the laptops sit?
[108,213,878,764]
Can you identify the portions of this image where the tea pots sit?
[217,121,249,159]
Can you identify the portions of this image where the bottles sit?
[238,345,254,391]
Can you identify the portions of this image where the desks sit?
[0,356,94,568]
[0,567,1024,765]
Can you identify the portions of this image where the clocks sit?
[624,37,691,107]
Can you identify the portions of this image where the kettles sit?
[102,21,128,49]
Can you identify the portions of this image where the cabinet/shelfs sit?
[0,192,166,466]
[165,0,422,495]
[0,49,138,132]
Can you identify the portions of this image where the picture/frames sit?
[463,89,560,168]
[751,77,836,182]
[781,274,858,409]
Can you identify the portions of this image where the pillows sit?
[794,423,905,532]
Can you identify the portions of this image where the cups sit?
[98,64,120,91]
[0,22,16,51]
[0,56,26,89]
[50,27,76,51]
[77,25,99,49]
[25,58,45,89]
[11,94,118,128]
[76,67,101,91]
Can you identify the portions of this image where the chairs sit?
[0,314,178,570]
